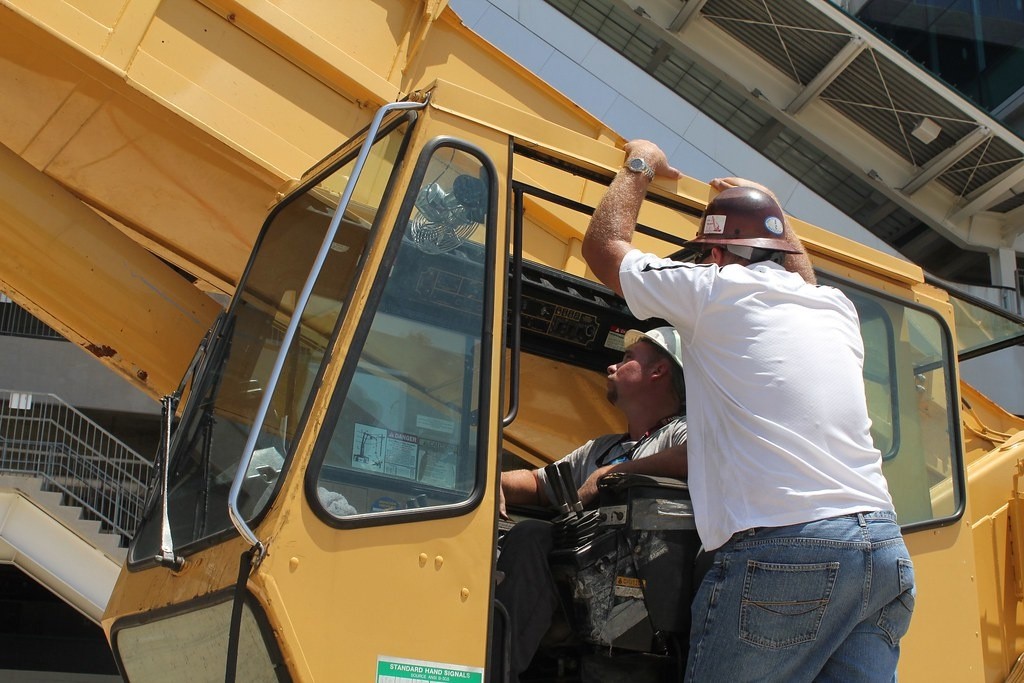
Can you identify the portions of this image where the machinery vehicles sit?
[0,1,1024,683]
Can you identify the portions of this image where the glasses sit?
[595,434,652,468]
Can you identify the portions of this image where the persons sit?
[580,140,917,683]
[499,326,688,683]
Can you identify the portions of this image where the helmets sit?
[683,186,803,254]
[624,326,683,368]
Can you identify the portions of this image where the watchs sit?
[623,157,655,182]
